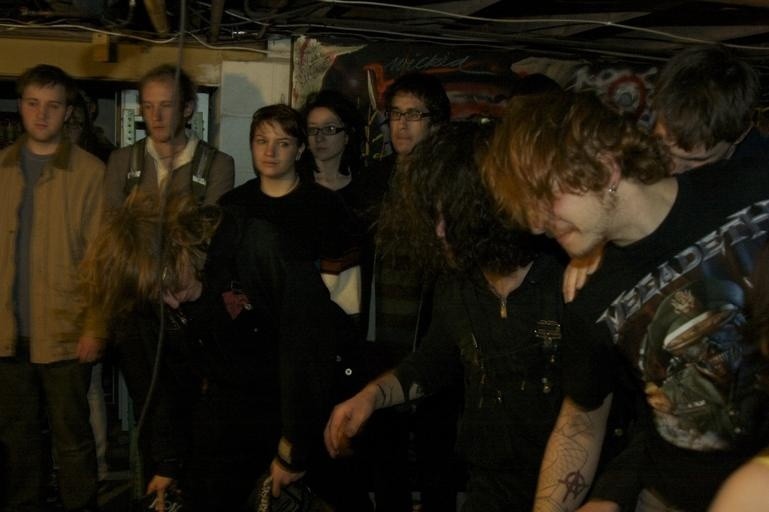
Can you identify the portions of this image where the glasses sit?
[384,108,433,121]
[305,125,346,136]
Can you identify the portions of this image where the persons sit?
[0,61,768,511]
